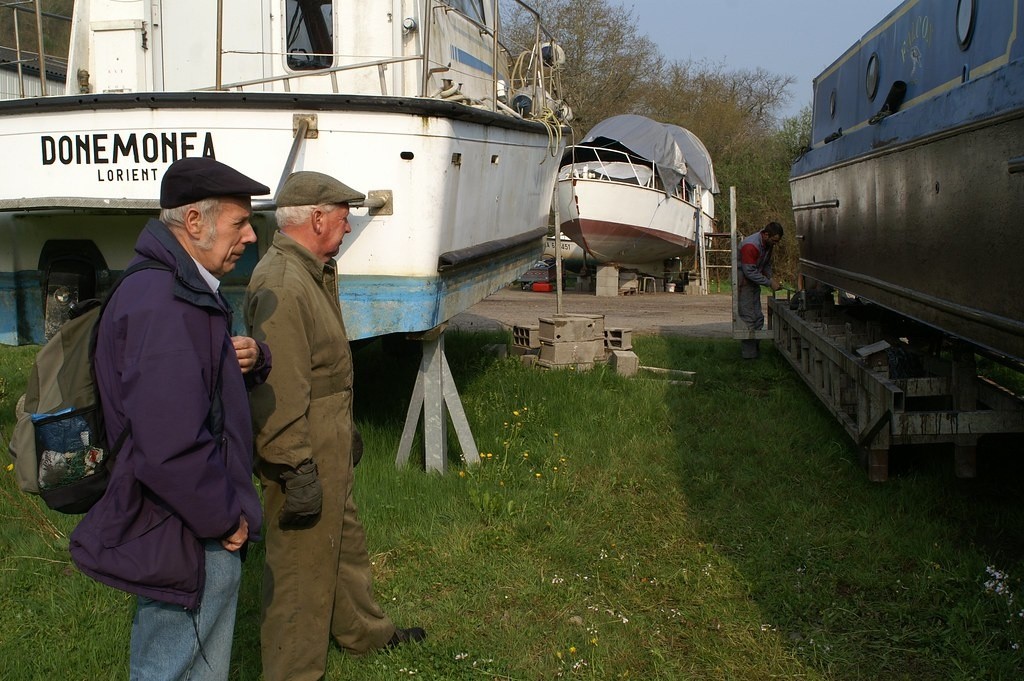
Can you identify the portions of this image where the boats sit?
[543,232,596,262]
[1,1,573,342]
[558,114,721,279]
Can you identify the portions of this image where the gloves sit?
[353,427,363,467]
[277,462,324,524]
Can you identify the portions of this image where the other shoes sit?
[378,627,426,655]
[742,350,761,358]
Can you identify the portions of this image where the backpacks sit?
[7,259,224,516]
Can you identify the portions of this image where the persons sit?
[69,157,272,681]
[737,222,784,358]
[243,171,427,681]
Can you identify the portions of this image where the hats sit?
[276,170,365,206]
[160,157,269,209]
